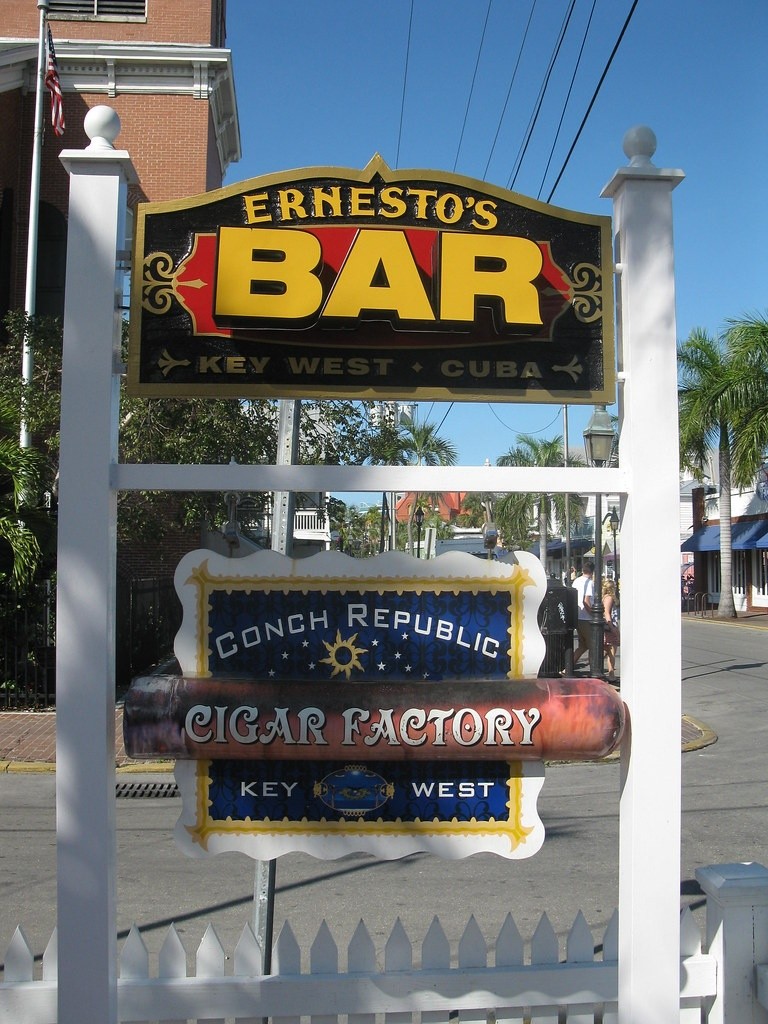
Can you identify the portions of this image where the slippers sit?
[608,678,620,683]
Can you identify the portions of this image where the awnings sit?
[526,539,620,558]
[681,519,768,552]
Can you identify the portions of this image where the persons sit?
[558,561,620,681]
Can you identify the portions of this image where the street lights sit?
[581,406,614,680]
[414,506,424,558]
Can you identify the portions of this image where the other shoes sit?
[559,671,566,677]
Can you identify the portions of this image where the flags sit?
[45,21,66,137]
